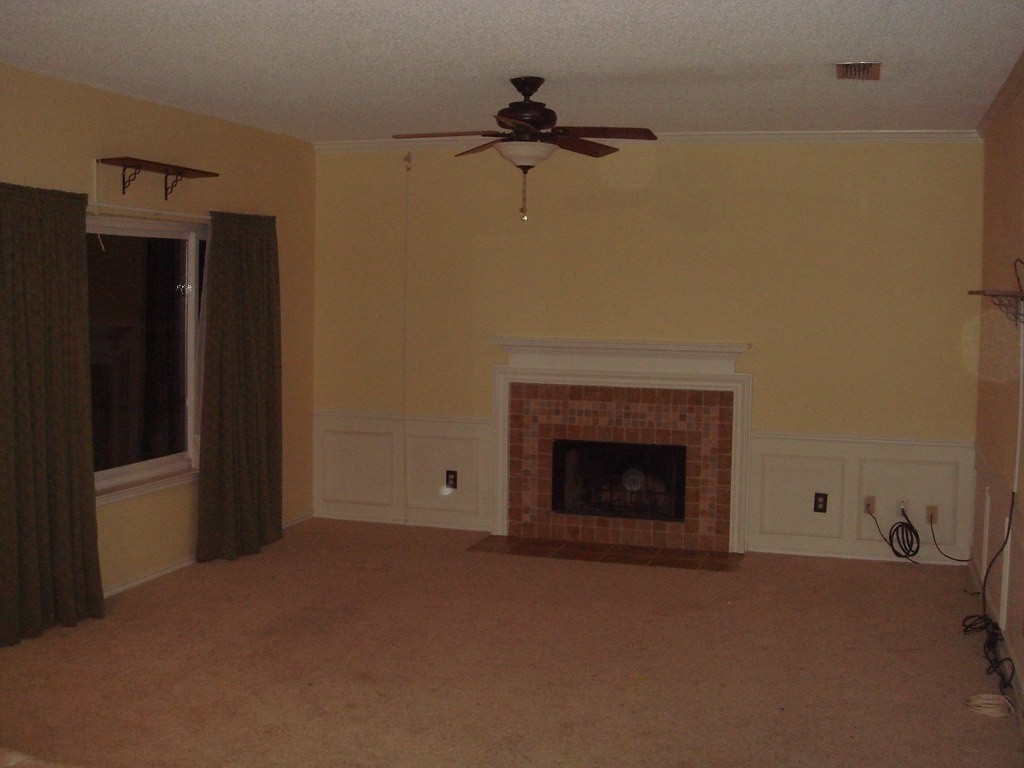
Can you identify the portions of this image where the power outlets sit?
[896,499,908,517]
[446,470,457,489]
[813,492,828,513]
[863,494,876,515]
[926,505,939,525]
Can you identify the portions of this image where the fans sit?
[392,77,658,159]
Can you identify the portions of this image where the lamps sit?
[493,142,562,222]
